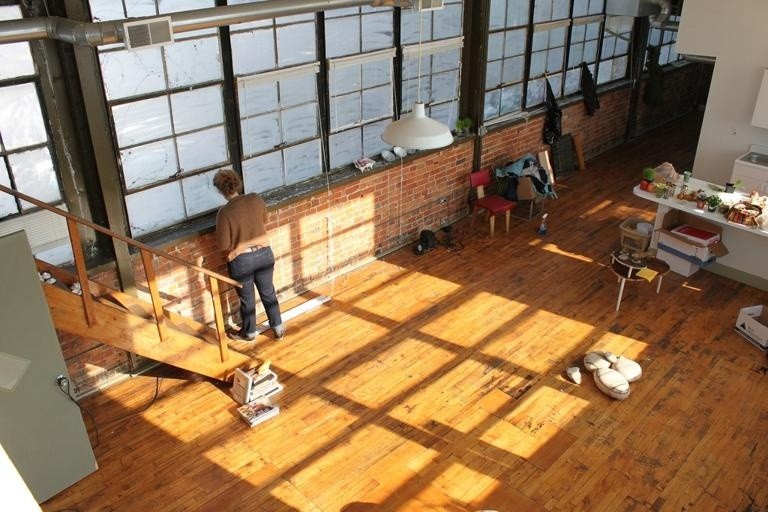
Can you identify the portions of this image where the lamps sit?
[381,0,455,151]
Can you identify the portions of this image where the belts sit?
[242,246,263,254]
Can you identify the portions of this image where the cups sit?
[684,170,692,181]
[656,181,676,199]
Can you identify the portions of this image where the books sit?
[231,362,284,426]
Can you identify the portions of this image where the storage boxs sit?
[651,227,702,278]
[733,305,768,353]
[671,224,730,269]
[621,230,650,253]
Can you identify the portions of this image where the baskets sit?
[618,215,653,252]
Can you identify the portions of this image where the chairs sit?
[469,170,512,237]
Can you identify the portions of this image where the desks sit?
[632,173,768,272]
[608,253,669,312]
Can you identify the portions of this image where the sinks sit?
[748,154,768,165]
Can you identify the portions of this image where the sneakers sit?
[274,325,287,341]
[228,330,256,343]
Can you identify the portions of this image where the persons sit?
[213,169,286,343]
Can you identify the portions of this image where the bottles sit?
[682,184,688,194]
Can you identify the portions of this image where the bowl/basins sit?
[394,147,407,157]
[382,150,395,161]
